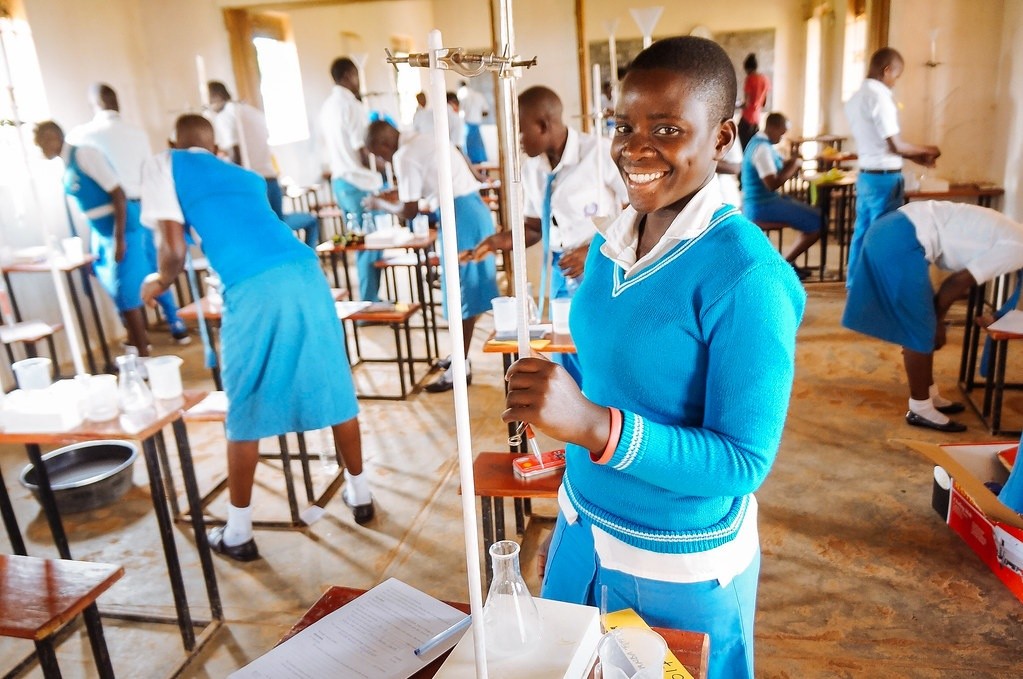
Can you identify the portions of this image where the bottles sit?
[482,540,543,679]
[347,212,374,234]
[115,353,153,412]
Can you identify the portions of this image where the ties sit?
[536,174,557,323]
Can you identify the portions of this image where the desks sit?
[0,136,1023,678]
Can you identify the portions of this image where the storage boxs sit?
[889,439,1023,603]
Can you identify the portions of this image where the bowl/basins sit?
[18,439,138,513]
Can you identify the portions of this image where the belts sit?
[859,168,901,174]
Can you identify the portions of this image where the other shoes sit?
[120,337,153,352]
[786,259,811,280]
[905,411,967,432]
[935,401,966,414]
[208,523,258,562]
[438,355,471,369]
[422,371,472,392]
[342,488,374,524]
[173,330,192,344]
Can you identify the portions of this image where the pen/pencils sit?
[522,423,544,467]
[414,614,471,656]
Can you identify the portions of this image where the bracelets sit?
[589,404,623,465]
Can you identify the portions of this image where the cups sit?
[376,214,392,231]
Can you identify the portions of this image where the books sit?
[229,577,472,679]
[431,592,600,679]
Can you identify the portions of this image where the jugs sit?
[11,357,52,391]
[78,374,120,422]
[145,355,185,399]
[62,237,82,263]
[596,627,668,679]
[412,215,429,238]
[551,299,570,334]
[491,296,517,332]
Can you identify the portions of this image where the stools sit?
[758,222,788,254]
[0,553,124,679]
[458,452,563,589]
[318,207,344,236]
[341,301,421,400]
[183,391,304,530]
[174,258,221,328]
[0,318,61,380]
[372,253,448,329]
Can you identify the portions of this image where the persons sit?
[841,198,1023,432]
[735,53,770,150]
[31,55,631,393]
[717,116,744,210]
[137,113,374,561]
[502,35,808,679]
[740,110,828,281]
[845,47,942,288]
